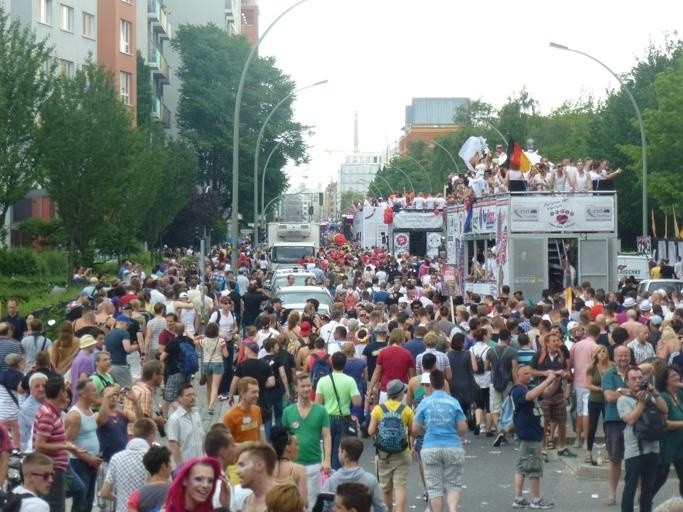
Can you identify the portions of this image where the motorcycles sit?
[37,319,56,340]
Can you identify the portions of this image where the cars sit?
[280,304,330,322]
[268,263,306,277]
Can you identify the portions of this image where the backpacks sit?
[173,337,199,375]
[374,404,408,453]
[625,393,666,455]
[311,353,331,390]
[472,346,519,434]
[0,492,33,512]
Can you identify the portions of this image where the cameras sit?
[639,379,649,390]
[119,387,127,393]
[154,408,166,437]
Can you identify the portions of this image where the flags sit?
[504,132,530,172]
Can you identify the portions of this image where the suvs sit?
[265,270,318,289]
[269,286,333,315]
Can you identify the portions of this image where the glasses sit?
[32,473,53,480]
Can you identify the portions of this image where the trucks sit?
[385,209,443,259]
[442,189,619,307]
[267,222,320,264]
[349,206,388,251]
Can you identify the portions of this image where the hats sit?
[622,298,651,311]
[386,380,407,397]
[567,321,579,331]
[649,315,662,325]
[5,353,22,365]
[79,334,97,349]
[116,315,133,324]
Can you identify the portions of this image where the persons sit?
[2,241,680,509]
[344,191,448,214]
[449,146,622,203]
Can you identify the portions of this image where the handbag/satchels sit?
[200,373,207,384]
[341,419,357,436]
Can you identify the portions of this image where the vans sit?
[617,254,650,284]
[637,279,683,318]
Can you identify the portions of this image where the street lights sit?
[383,162,414,192]
[260,123,318,235]
[348,172,393,200]
[467,114,508,148]
[416,136,459,174]
[273,174,308,219]
[395,151,432,192]
[253,76,331,252]
[549,41,649,237]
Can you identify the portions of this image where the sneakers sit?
[558,448,576,456]
[512,497,554,509]
